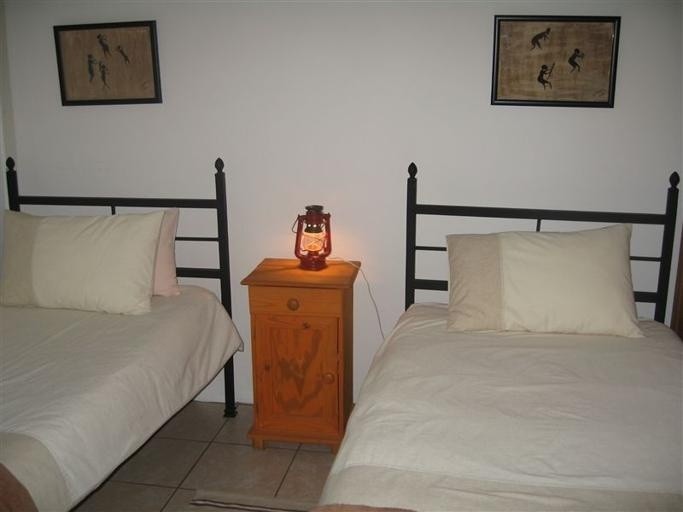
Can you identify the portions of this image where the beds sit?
[0,157,237,512]
[318,162,683,511]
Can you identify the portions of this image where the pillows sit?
[445,221,644,338]
[1,208,183,313]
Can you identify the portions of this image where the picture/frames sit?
[54,20,163,105]
[491,15,621,108]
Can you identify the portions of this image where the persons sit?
[98,61,106,92]
[567,48,583,73]
[95,32,110,58]
[86,53,97,84]
[529,27,551,50]
[116,45,130,66]
[536,64,552,90]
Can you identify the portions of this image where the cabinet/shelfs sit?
[240,258,361,455]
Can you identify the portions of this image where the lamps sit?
[292,206,331,271]
